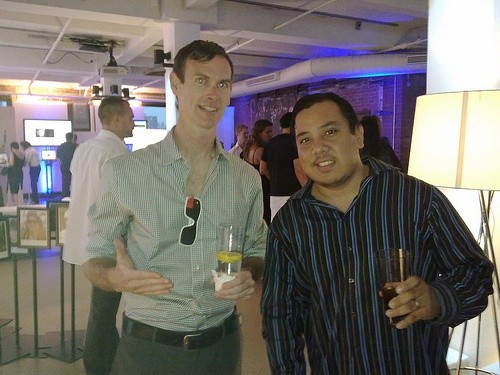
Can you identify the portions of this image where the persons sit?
[242,119,273,226]
[56,132,79,202]
[20,141,41,204]
[231,137,237,148]
[73,133,78,145]
[62,96,136,375]
[362,115,390,145]
[259,91,496,375]
[4,142,25,206]
[359,119,405,174]
[227,124,250,160]
[82,39,268,375]
[259,112,310,224]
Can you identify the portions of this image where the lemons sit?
[218,251,242,262]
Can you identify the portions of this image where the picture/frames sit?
[0,215,17,261]
[16,206,51,248]
[46,200,70,246]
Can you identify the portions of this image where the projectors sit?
[99,66,127,76]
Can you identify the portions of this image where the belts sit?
[122,305,244,350]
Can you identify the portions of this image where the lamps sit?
[406,90,500,375]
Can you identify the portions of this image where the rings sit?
[410,299,420,310]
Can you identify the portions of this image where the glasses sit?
[179,196,202,247]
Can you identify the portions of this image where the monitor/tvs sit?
[41,150,57,161]
[23,119,73,147]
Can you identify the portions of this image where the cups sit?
[216,225,245,276]
[380,248,410,328]
